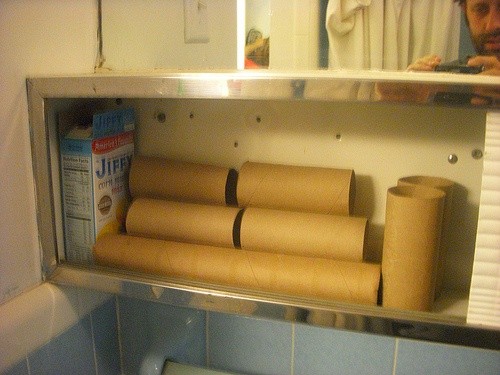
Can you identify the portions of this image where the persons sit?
[400,0,500,79]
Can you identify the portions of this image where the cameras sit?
[435,54,492,106]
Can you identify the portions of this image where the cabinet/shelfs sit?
[25,69,500,352]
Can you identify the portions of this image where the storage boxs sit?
[58,107,136,265]
